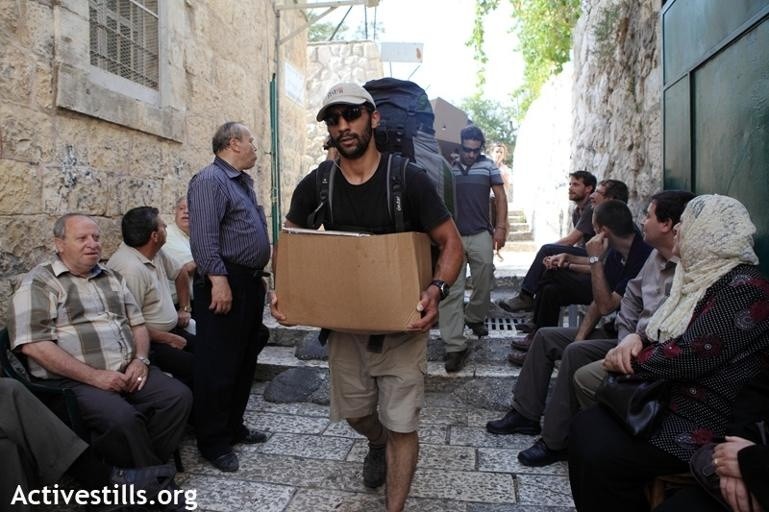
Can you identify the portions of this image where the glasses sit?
[461,144,481,153]
[324,106,364,125]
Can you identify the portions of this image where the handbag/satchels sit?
[594,372,663,436]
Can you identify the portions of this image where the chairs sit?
[1,327,186,488]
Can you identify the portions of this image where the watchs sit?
[134,354,152,370]
[430,279,449,302]
[179,304,193,312]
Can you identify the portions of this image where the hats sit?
[317,84,375,121]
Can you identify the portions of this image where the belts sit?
[227,266,270,280]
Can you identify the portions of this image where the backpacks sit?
[318,78,459,232]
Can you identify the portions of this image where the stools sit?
[641,471,699,511]
[557,299,622,329]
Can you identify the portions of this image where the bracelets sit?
[495,226,507,234]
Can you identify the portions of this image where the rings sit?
[136,377,143,384]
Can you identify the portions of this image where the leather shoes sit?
[487,408,541,434]
[197,441,239,472]
[518,438,567,466]
[500,292,534,364]
[228,430,267,445]
[78,464,176,511]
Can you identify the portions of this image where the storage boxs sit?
[429,96,469,156]
[270,227,435,334]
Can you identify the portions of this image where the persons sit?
[104,205,199,436]
[485,168,768,512]
[187,116,274,474]
[437,127,508,374]
[163,196,201,304]
[5,213,196,511]
[0,376,179,511]
[267,79,466,511]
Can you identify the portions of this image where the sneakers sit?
[362,441,385,489]
[464,318,487,334]
[445,349,466,370]
